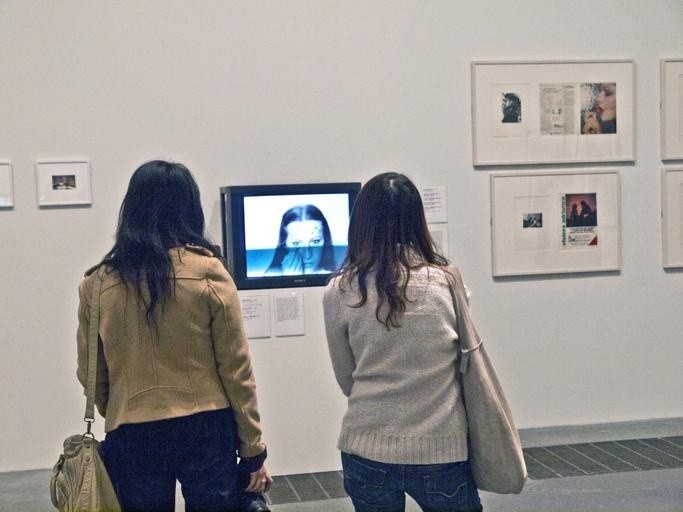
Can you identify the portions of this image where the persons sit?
[73,162,273,512]
[570,199,596,227]
[265,206,335,277]
[580,81,617,136]
[321,171,490,512]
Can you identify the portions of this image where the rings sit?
[261,476,268,485]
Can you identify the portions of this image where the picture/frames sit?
[490,169,622,277]
[471,59,636,168]
[35,159,93,208]
[660,167,683,269]
[659,58,683,161]
[0,161,13,207]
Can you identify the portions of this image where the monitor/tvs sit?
[219,181,363,291]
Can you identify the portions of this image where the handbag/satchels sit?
[50,434,123,512]
[442,268,527,494]
